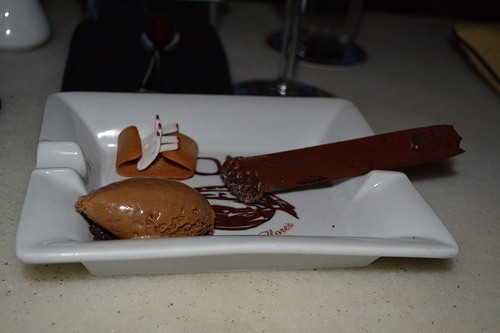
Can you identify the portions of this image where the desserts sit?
[74,113,216,239]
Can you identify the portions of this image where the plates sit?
[15,92,459,275]
